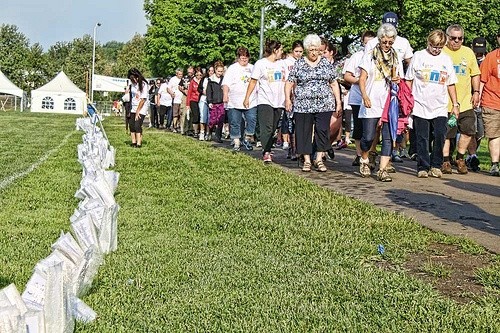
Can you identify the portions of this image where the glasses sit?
[446,34,463,41]
[380,39,394,45]
[428,42,444,49]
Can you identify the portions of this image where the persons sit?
[114,11,500,182]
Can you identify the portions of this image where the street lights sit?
[91,22,102,102]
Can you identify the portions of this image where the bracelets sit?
[473,90,480,94]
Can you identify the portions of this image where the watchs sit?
[454,102,460,107]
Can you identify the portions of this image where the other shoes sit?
[417,170,428,178]
[351,155,362,166]
[313,159,327,172]
[441,161,452,174]
[489,164,500,176]
[408,153,418,161]
[428,167,442,178]
[302,161,312,172]
[368,150,378,163]
[147,123,353,163]
[378,162,396,173]
[399,151,407,158]
[456,160,468,174]
[359,159,371,178]
[391,155,403,163]
[128,142,141,149]
[377,170,393,182]
[465,154,480,173]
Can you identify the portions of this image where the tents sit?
[0,68,89,115]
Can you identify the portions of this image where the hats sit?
[472,37,487,54]
